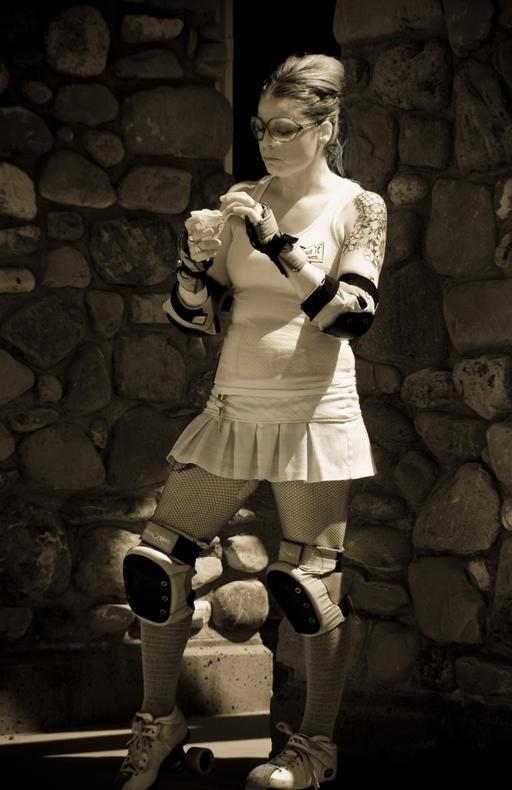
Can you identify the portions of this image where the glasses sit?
[247,113,325,143]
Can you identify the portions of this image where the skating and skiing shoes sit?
[107,703,218,790]
[243,721,340,790]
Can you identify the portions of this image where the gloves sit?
[181,213,225,263]
[218,191,265,226]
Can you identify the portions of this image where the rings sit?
[195,244,203,252]
[187,234,196,246]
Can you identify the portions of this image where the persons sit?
[120,46,389,790]
